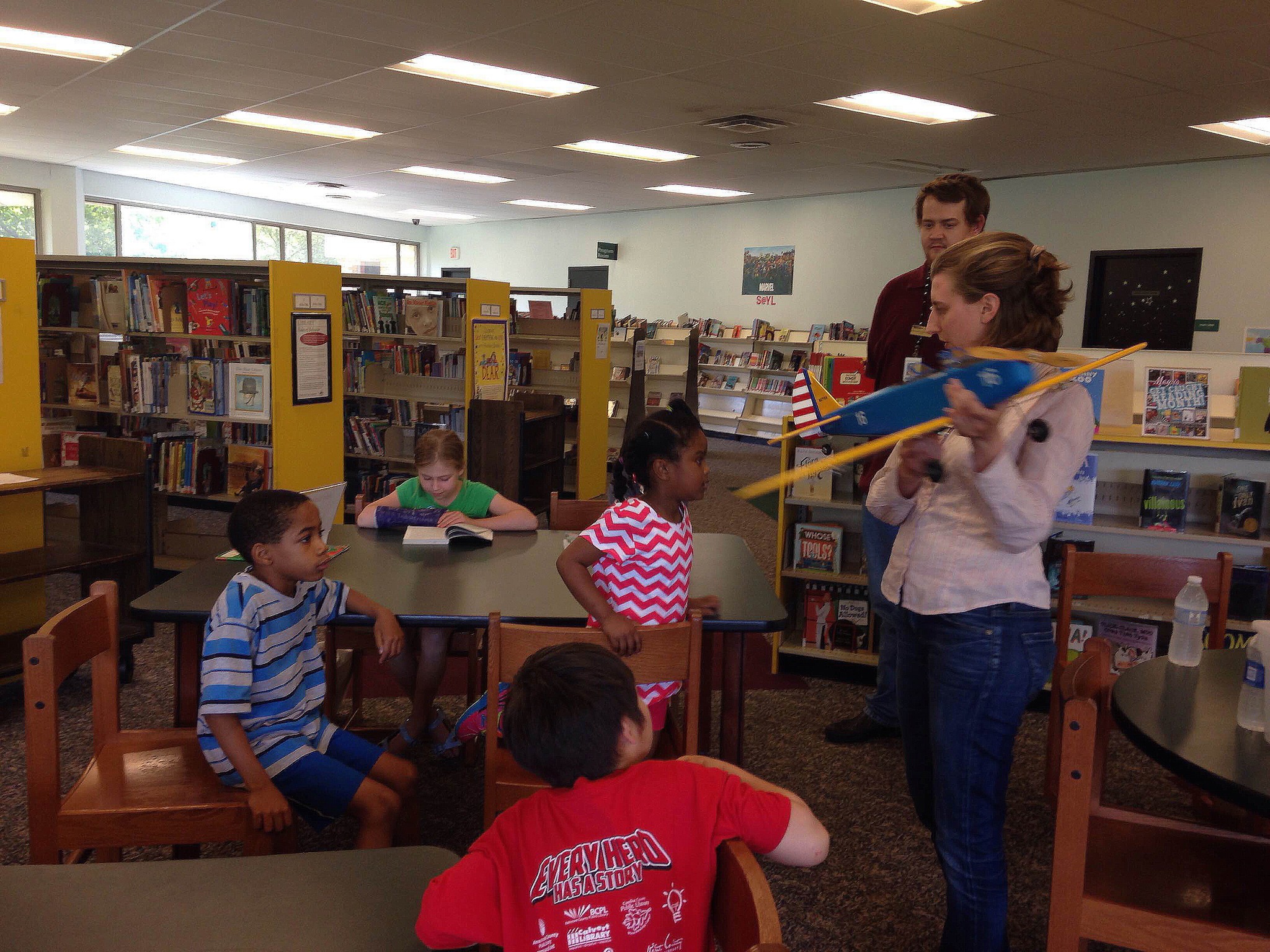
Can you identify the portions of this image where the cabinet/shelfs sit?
[506,288,607,499]
[606,327,645,474]
[648,326,701,425]
[642,325,828,443]
[466,391,566,518]
[338,272,511,517]
[767,414,1270,677]
[0,434,156,710]
[35,254,344,555]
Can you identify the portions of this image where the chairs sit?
[479,612,702,835]
[1052,543,1233,669]
[21,577,290,864]
[547,491,611,533]
[320,624,365,733]
[1047,637,1270,952]
[707,833,792,952]
[354,495,477,720]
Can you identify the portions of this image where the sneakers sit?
[456,682,512,743]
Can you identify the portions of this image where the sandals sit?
[425,705,463,761]
[375,716,425,758]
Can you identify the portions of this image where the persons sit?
[196,489,417,846]
[449,398,720,742]
[866,232,1094,949]
[823,170,990,740]
[354,428,540,528]
[415,642,830,952]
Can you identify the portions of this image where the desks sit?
[1107,647,1270,822]
[126,526,790,765]
[0,846,482,951]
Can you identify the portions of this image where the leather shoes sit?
[821,714,894,743]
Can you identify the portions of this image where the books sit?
[788,325,1270,694]
[505,299,869,464]
[214,542,349,562]
[39,269,268,582]
[338,293,460,506]
[402,524,495,544]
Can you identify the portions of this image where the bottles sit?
[1235,622,1270,742]
[1166,573,1209,667]
